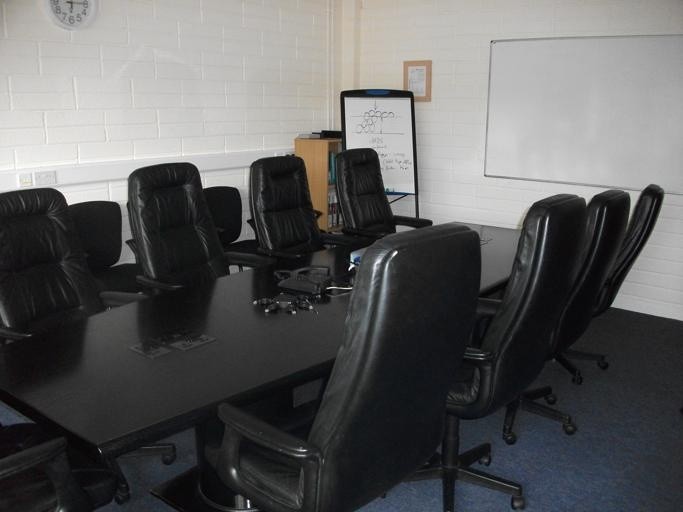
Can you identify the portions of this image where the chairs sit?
[1,402,118,511]
[214,221,483,511]
[403,184,665,511]
[1,188,111,346]
[331,148,435,240]
[248,154,368,260]
[127,162,280,296]
[67,200,142,310]
[200,185,258,255]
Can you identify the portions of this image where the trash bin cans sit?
[0,451,57,512]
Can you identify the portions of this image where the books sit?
[329,151,343,227]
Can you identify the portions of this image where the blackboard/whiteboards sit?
[483,33,683,196]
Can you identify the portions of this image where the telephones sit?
[276,265,333,299]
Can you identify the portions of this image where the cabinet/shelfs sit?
[296,140,344,234]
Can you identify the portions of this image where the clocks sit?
[44,0,98,29]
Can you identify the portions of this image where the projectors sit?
[350,247,369,267]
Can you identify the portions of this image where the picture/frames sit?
[403,60,432,102]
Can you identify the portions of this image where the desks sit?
[1,218,522,501]
